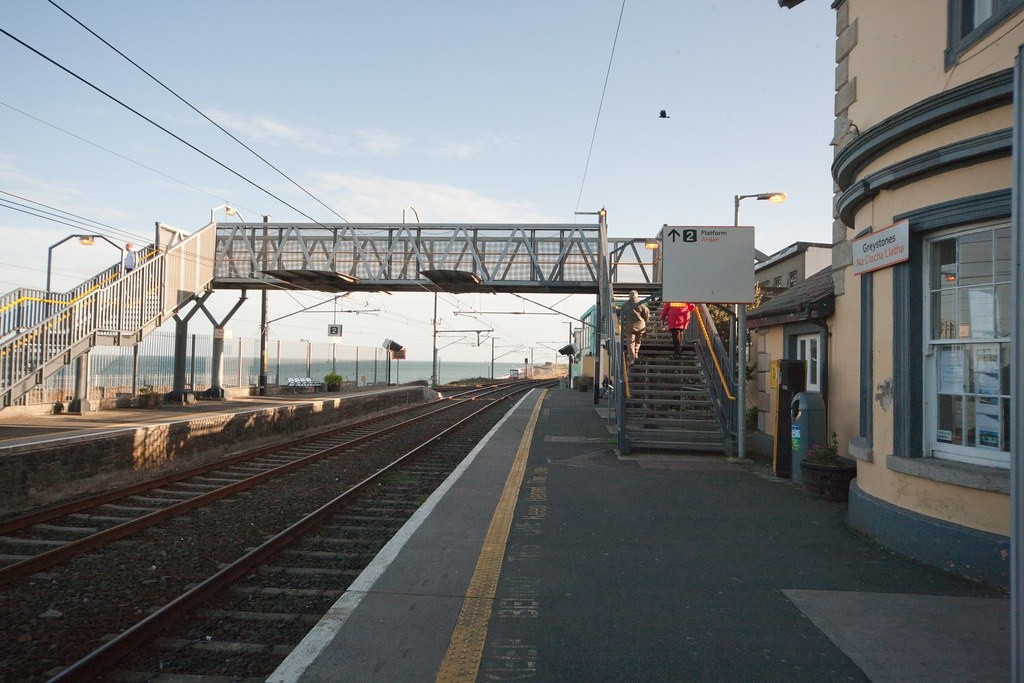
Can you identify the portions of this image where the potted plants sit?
[139,387,163,408]
[325,375,343,392]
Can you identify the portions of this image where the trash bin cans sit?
[790,392,826,482]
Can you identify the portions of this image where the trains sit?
[510,368,523,378]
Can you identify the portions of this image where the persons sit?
[620,287,651,368]
[599,374,612,398]
[124,242,139,274]
[661,302,696,360]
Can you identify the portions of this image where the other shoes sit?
[630,356,639,367]
[673,350,682,358]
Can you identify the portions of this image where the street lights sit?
[735,191,786,457]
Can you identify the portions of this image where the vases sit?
[801,460,857,502]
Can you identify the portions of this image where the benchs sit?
[285,377,322,394]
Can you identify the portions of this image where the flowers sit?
[807,432,839,464]
